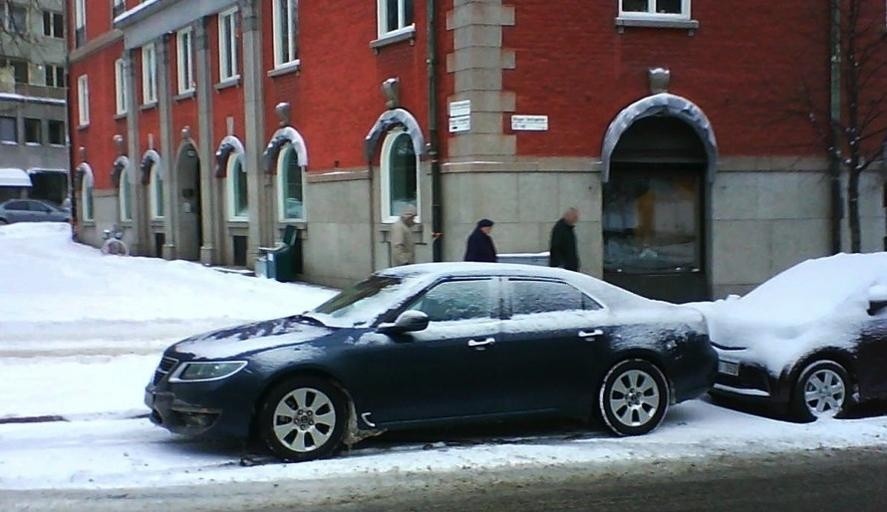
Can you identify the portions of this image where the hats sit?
[477,220,493,227]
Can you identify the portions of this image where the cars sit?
[0,199,69,225]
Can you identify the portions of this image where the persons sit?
[548,206,581,271]
[461,218,499,263]
[389,204,416,266]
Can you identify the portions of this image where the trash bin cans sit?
[267,225,297,282]
